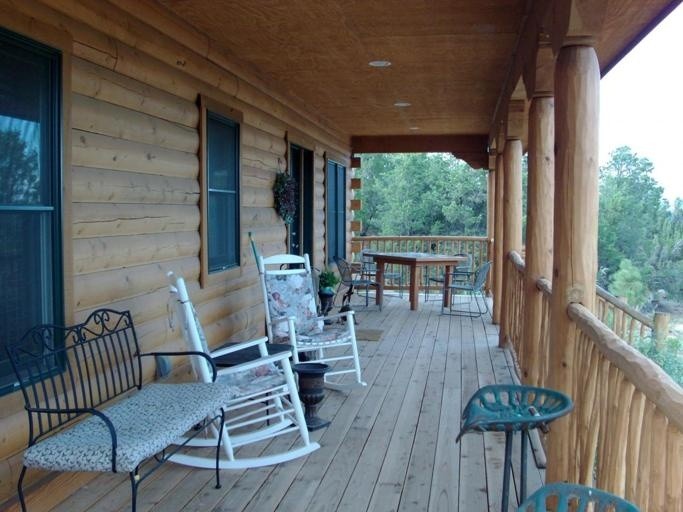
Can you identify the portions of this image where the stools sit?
[454,383,576,511]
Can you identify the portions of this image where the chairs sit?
[332,248,493,319]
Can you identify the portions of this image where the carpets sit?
[322,327,383,342]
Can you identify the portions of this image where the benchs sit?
[3,307,233,512]
